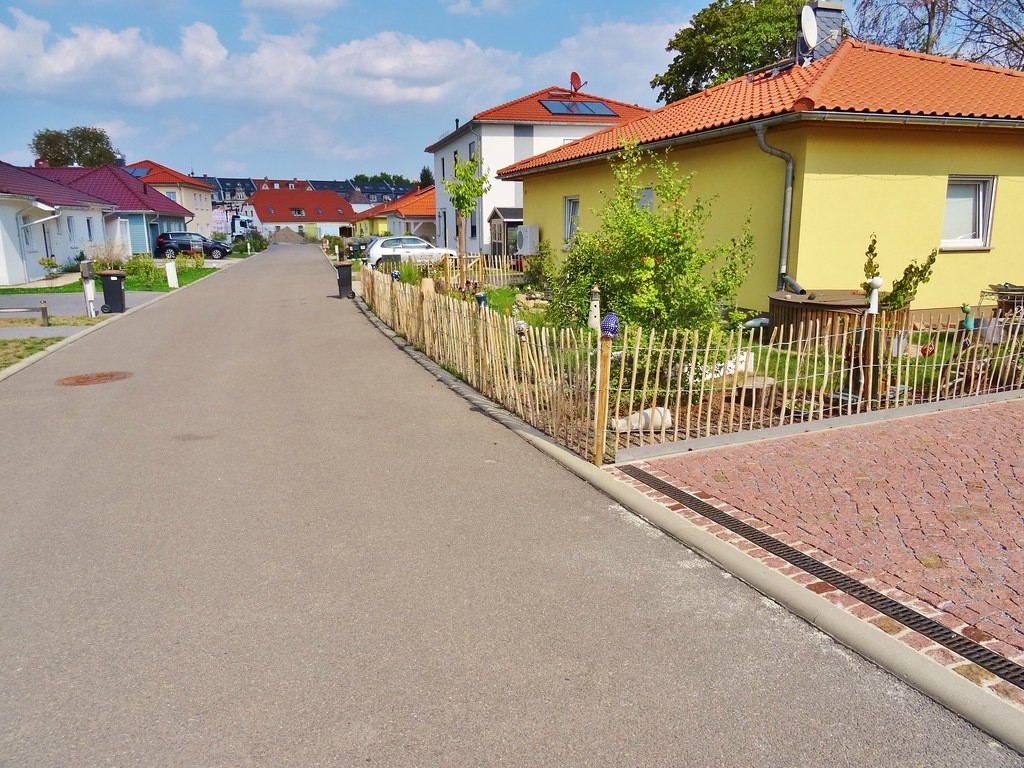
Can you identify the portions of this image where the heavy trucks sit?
[213,210,255,242]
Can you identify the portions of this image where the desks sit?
[976,289,1024,314]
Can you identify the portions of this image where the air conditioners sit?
[517,224,539,256]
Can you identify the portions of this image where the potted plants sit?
[38,256,59,277]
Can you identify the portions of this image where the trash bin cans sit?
[334,260,355,300]
[95,270,127,313]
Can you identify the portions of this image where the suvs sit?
[364,236,457,272]
[156,231,232,260]
